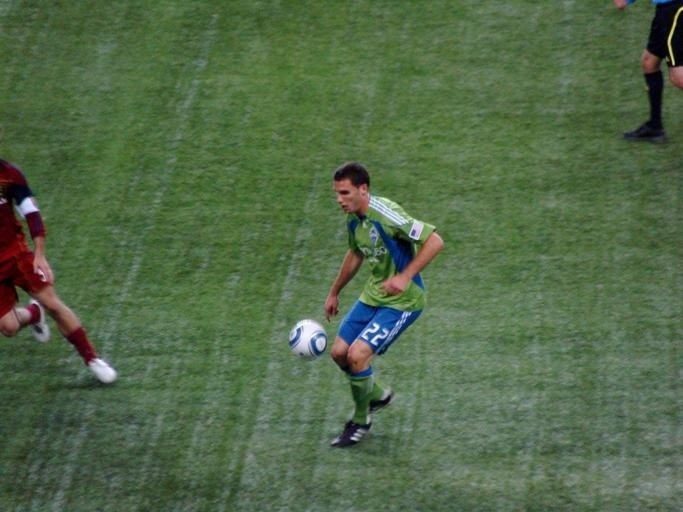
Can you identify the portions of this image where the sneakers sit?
[329,415,373,447]
[622,120,666,144]
[27,296,49,343]
[345,385,393,422]
[78,355,117,384]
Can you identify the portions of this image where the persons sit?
[0,158,118,385]
[617,0,682,140]
[324,163,444,448]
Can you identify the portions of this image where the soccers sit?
[287,319,328,361]
